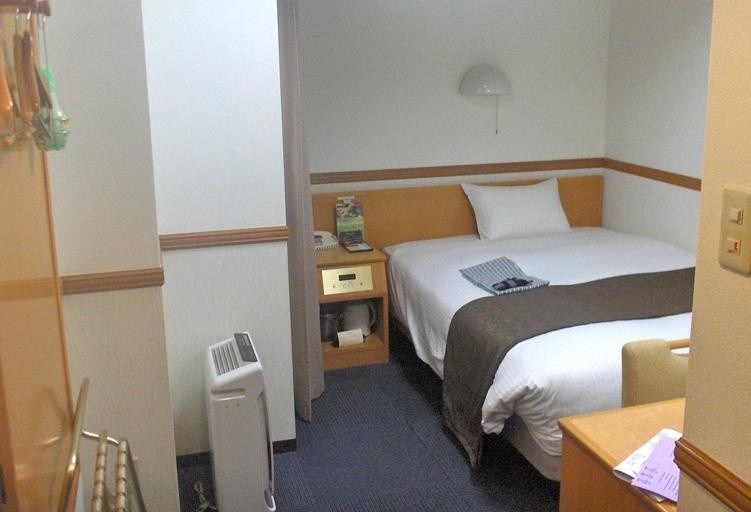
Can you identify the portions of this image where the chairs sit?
[622,337,689,407]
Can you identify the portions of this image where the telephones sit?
[313,231,337,249]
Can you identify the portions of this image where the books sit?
[334,195,366,246]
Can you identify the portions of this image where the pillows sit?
[460,177,573,239]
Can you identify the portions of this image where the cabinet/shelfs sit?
[557,398,687,510]
[0,0,82,512]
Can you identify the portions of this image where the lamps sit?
[459,63,513,96]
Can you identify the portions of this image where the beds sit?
[384,226,695,498]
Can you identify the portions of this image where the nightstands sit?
[314,239,390,371]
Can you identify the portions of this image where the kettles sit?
[340,301,377,336]
